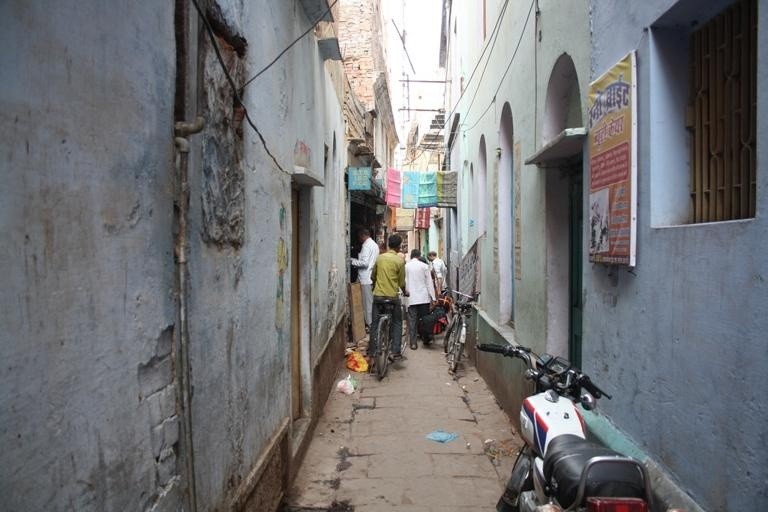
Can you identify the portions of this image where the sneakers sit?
[391,355,408,364]
[412,345,417,349]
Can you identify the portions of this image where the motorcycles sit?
[476,343,656,512]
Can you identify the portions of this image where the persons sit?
[350,230,379,333]
[398,249,448,301]
[400,249,437,350]
[367,235,409,373]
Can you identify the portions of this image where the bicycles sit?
[366,289,481,383]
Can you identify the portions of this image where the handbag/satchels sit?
[438,294,451,312]
[417,307,448,334]
